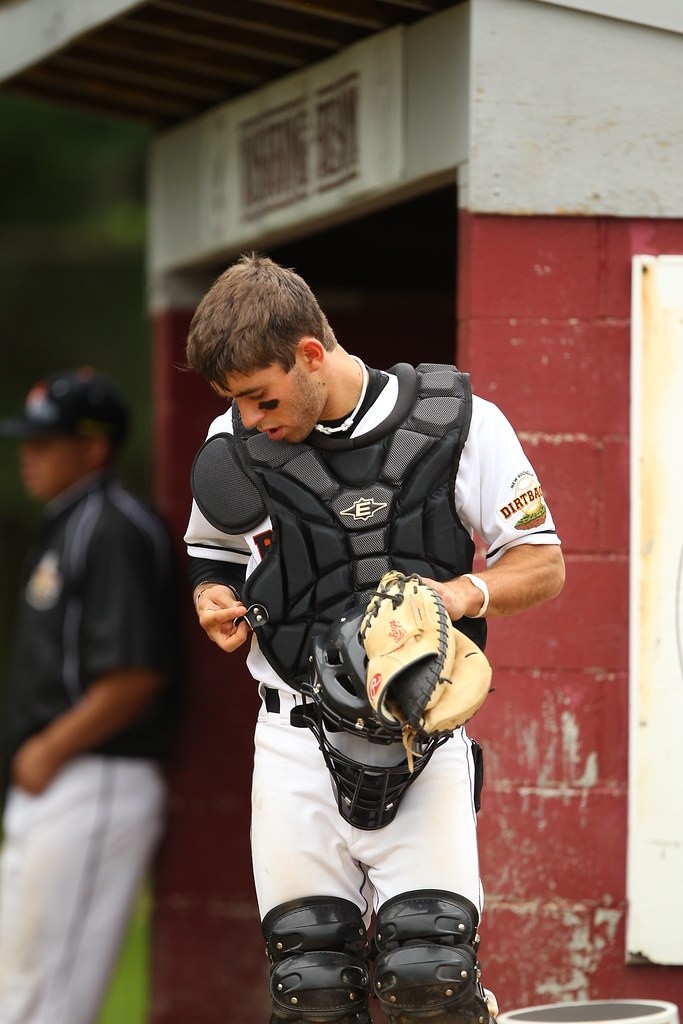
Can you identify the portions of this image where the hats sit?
[0,366,132,445]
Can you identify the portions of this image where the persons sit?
[182,255,568,1024]
[0,365,191,1024]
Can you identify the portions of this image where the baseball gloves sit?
[356,572,492,739]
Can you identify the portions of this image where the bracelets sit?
[196,589,206,604]
[460,574,489,618]
